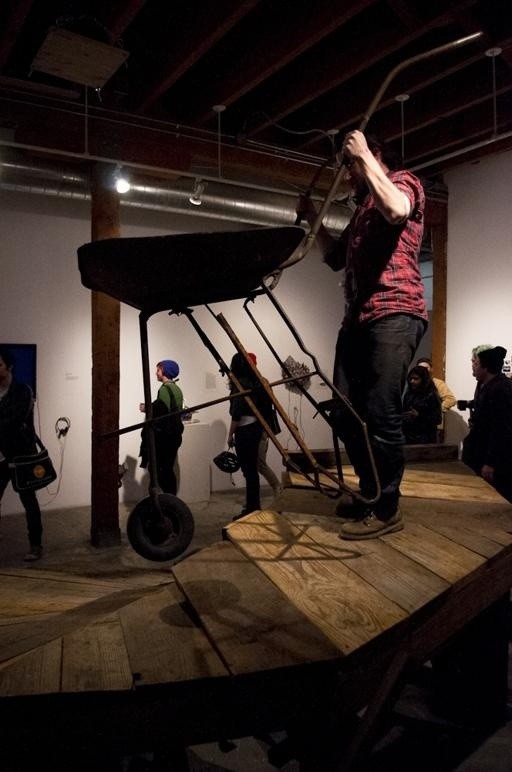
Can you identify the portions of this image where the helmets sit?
[248,352,256,365]
[212,451,240,473]
[478,345,507,374]
[162,360,179,380]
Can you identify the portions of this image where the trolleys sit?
[75,30,489,566]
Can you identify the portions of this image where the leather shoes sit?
[338,509,405,540]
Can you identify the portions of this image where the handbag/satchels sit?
[9,447,58,493]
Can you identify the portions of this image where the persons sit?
[412,356,458,443]
[293,116,429,542]
[1,353,46,561]
[139,360,184,495]
[248,354,282,496]
[458,345,493,424]
[401,367,440,445]
[462,345,511,503]
[228,350,272,522]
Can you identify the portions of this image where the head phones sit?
[55,416,71,438]
